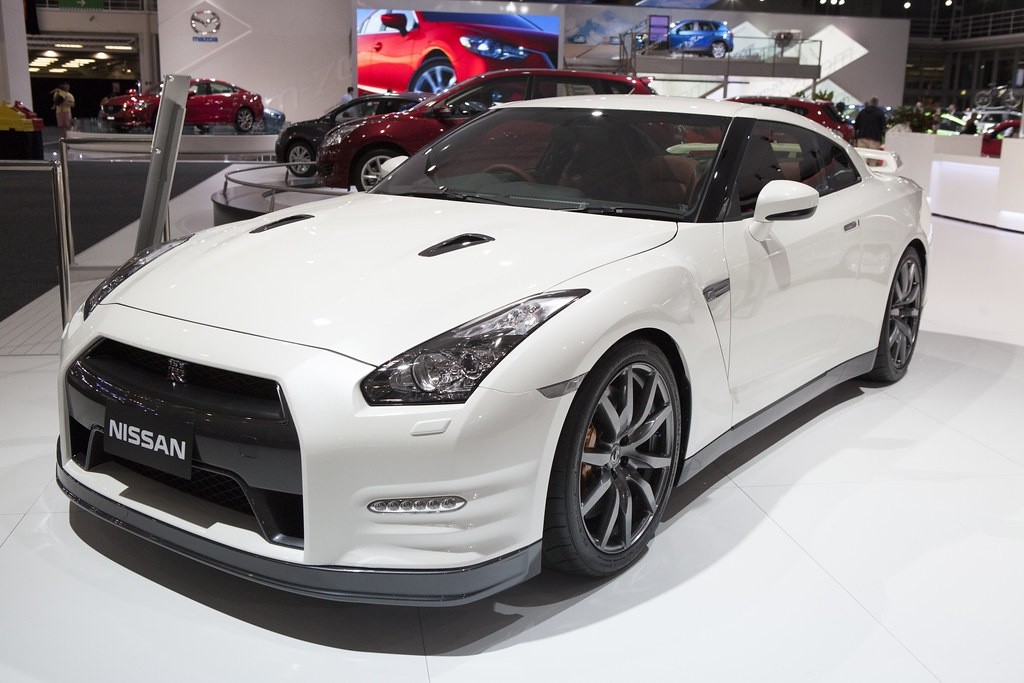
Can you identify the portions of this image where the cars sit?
[634,18,734,58]
[356,10,561,110]
[59,91,936,621]
[97,74,263,132]
[724,95,856,147]
[894,101,1022,140]
[251,108,285,133]
[275,92,439,179]
[0,100,45,134]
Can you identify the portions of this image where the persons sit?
[52,83,76,138]
[910,97,993,138]
[853,96,886,152]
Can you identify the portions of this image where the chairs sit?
[554,122,809,214]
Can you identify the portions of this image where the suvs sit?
[316,67,664,195]
